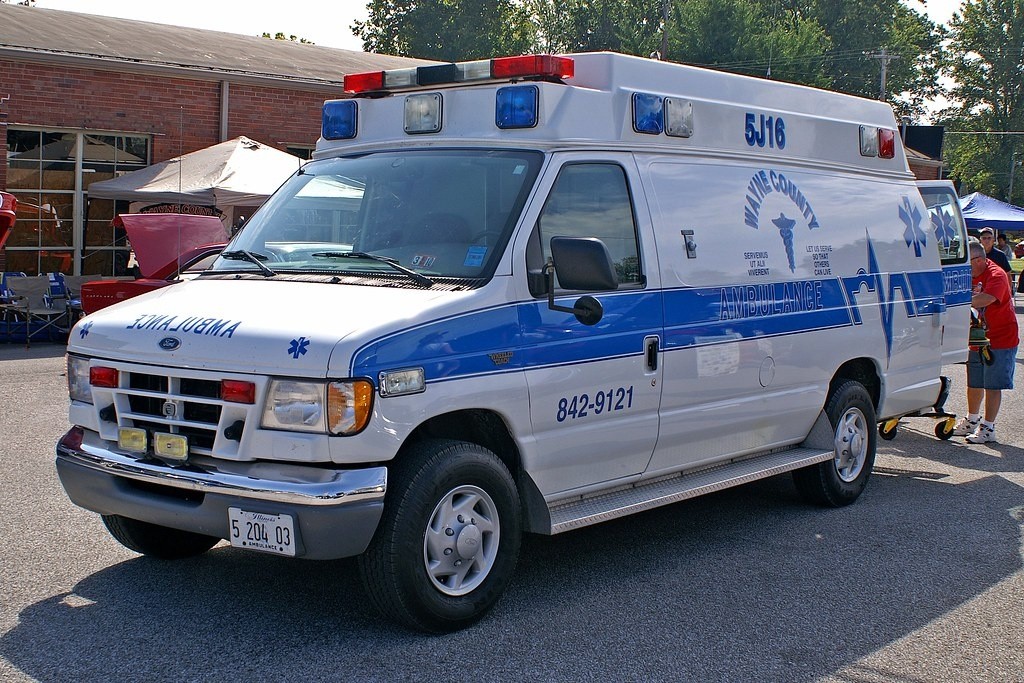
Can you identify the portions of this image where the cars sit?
[77,213,355,317]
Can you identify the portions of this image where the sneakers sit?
[965,423,996,444]
[953,416,983,435]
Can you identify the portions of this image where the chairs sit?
[406,212,471,250]
[7,276,70,348]
[63,274,103,329]
[0,272,51,343]
[488,211,544,269]
[38,272,73,335]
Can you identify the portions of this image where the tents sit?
[927,191,1024,245]
[10,133,145,169]
[88,135,366,243]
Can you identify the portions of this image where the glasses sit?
[981,237,992,240]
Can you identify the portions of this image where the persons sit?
[43,197,60,227]
[952,241,1020,445]
[938,227,1017,300]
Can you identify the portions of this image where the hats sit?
[997,234,1006,240]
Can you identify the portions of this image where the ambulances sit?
[53,51,972,638]
